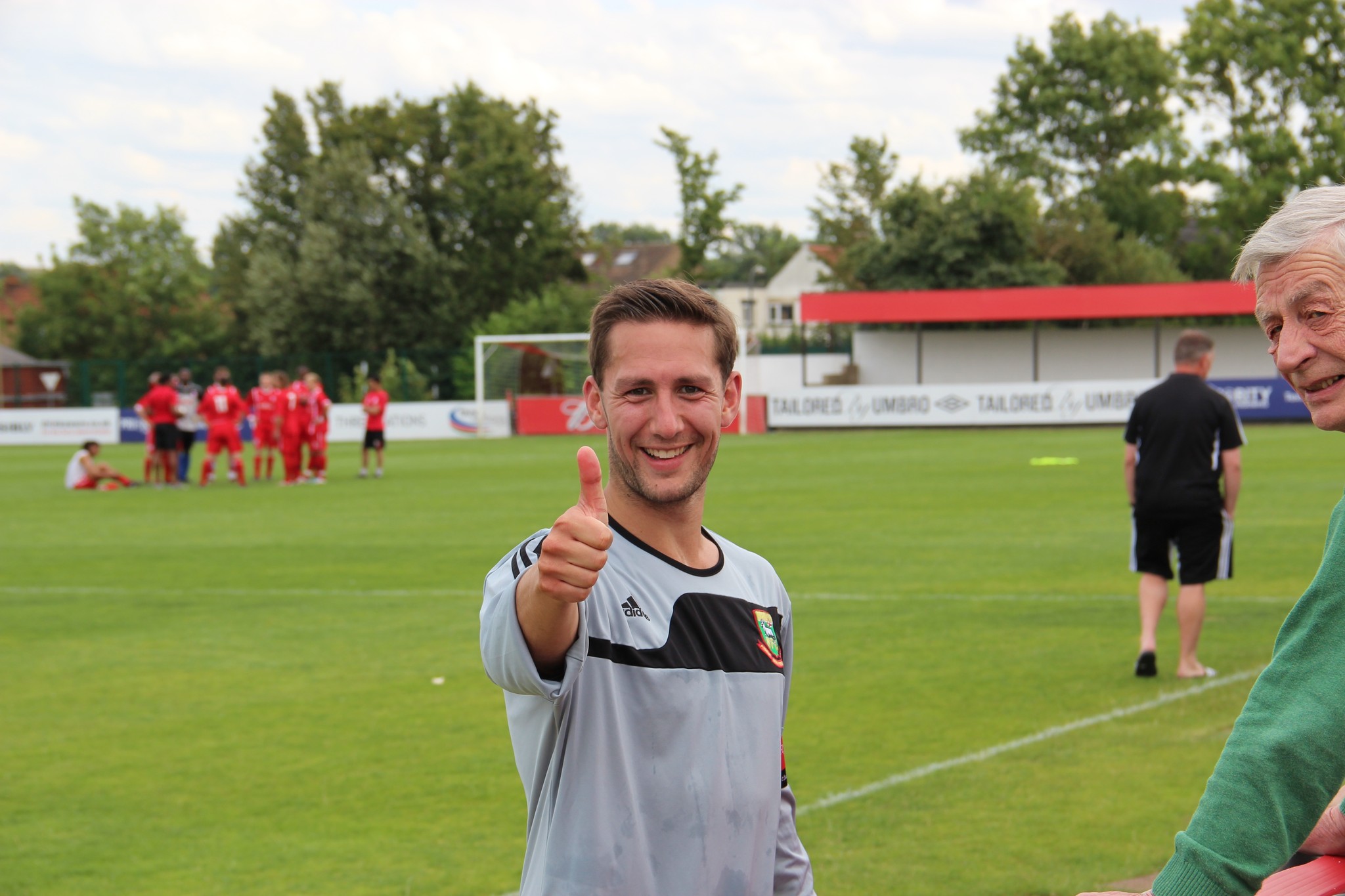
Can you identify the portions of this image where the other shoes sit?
[1136,652,1156,677]
[1178,666,1217,678]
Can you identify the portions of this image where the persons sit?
[356,374,388,477]
[1073,187,1345,896]
[65,442,138,489]
[479,277,818,895]
[1122,328,1246,681]
[135,365,332,486]
